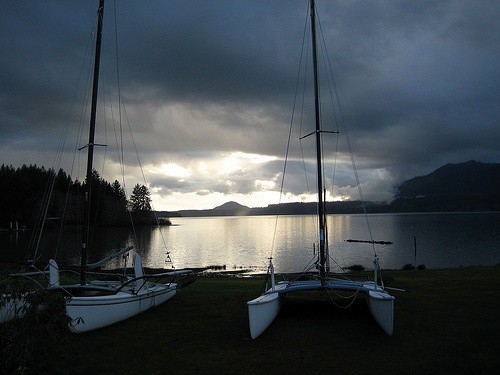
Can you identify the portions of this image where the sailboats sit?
[248,0,397,342]
[0,1,181,333]
